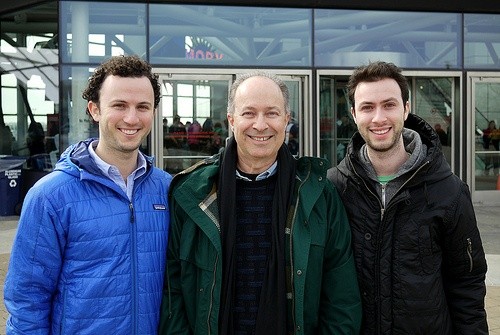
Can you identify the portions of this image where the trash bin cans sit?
[0,159,30,216]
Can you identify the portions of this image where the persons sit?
[3,56,173,335]
[482,121,500,177]
[163,115,229,157]
[433,124,447,146]
[327,62,489,335]
[0,122,59,172]
[284,110,358,166]
[157,74,361,335]
